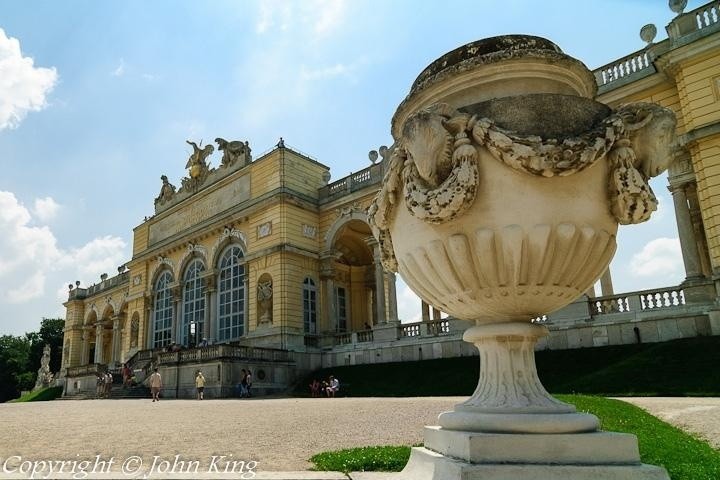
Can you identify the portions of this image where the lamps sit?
[190,320,196,344]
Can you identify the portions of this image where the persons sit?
[149,367,162,402]
[246,370,253,398]
[364,322,372,341]
[162,341,181,352]
[239,368,247,397]
[95,363,137,399]
[308,375,340,397]
[195,371,206,400]
[201,338,209,348]
